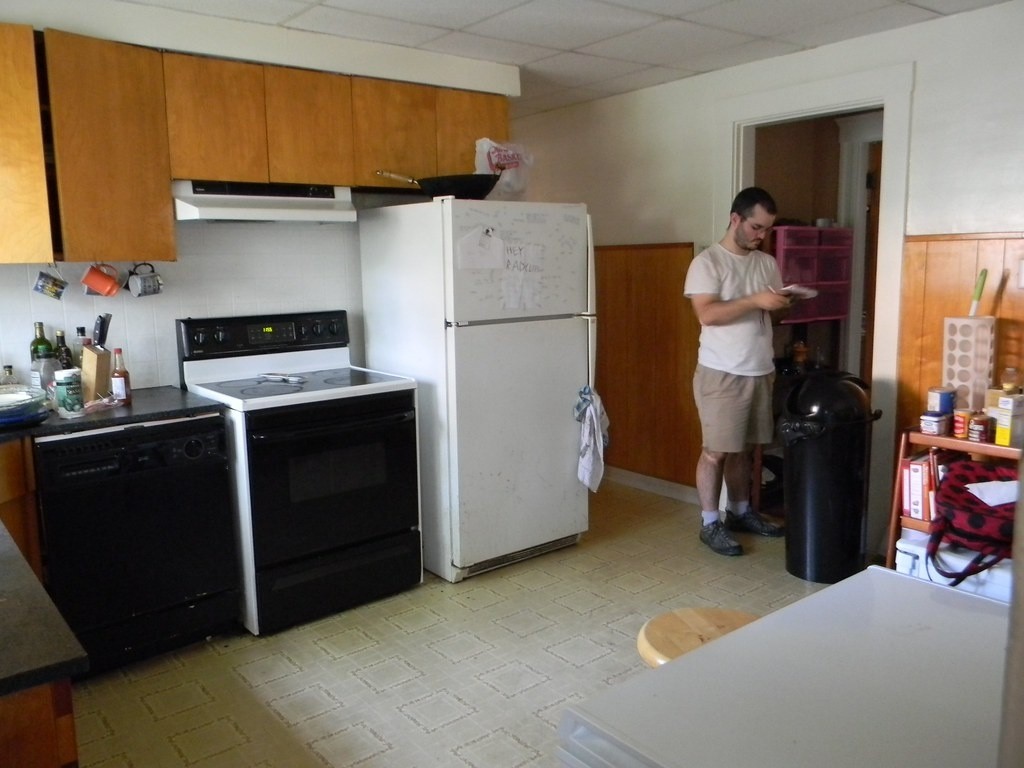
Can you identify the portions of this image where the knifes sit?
[93,313,112,351]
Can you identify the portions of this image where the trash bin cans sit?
[780,370,883,585]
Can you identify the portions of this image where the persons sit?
[682,187,800,555]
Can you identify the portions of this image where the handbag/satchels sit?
[924,460,1018,587]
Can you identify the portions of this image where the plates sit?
[0,408,49,427]
[0,384,46,413]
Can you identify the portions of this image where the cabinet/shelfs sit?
[161,49,351,186]
[888,431,1020,569]
[769,227,852,324]
[0,24,175,263]
[351,75,510,189]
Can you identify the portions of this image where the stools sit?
[636,607,760,668]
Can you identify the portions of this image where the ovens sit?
[224,389,423,637]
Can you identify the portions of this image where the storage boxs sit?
[994,394,1024,446]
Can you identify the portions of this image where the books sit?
[901,446,972,521]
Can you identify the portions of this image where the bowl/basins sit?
[811,217,832,226]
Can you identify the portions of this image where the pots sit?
[376,167,503,199]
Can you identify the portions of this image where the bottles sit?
[1000,367,1019,395]
[0,365,19,385]
[30,352,62,389]
[969,415,997,443]
[30,322,52,361]
[953,408,977,438]
[111,348,131,405]
[55,382,81,412]
[928,386,957,413]
[52,331,72,369]
[74,327,92,368]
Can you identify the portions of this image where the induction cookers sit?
[175,310,418,411]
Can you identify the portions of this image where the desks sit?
[554,564,1024,768]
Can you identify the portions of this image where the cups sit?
[81,264,120,297]
[123,263,164,297]
[33,267,69,300]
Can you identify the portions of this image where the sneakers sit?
[722,506,780,536]
[699,518,744,556]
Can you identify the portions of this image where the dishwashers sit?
[34,416,235,684]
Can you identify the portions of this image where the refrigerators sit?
[359,195,597,583]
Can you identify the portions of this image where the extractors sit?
[172,180,358,222]
